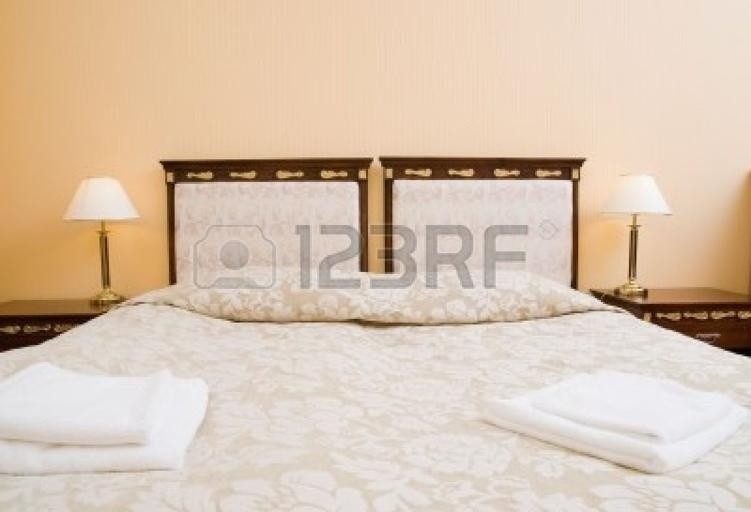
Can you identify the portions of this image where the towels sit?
[477,365,750,476]
[0,362,212,472]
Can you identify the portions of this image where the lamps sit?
[64,177,142,306]
[599,172,676,296]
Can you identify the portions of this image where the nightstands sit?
[0,298,108,353]
[593,288,751,356]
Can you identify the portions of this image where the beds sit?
[0,158,751,508]
